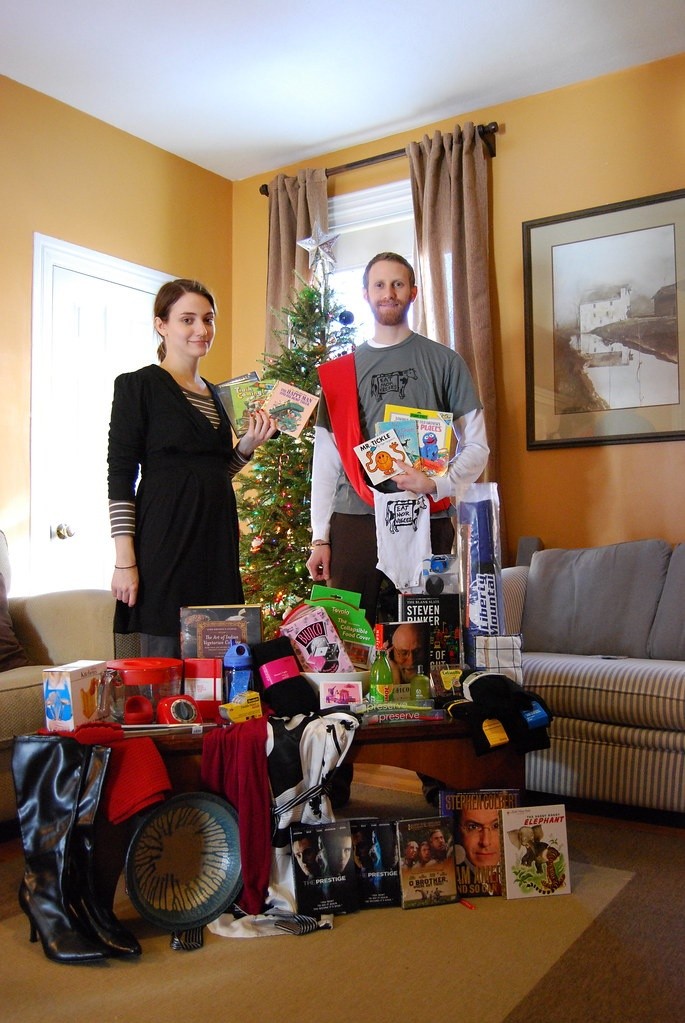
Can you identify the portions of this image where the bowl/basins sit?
[304,670,369,701]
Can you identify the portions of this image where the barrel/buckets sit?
[107,657,183,714]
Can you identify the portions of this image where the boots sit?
[9,733,144,966]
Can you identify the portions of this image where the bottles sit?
[411,664,430,700]
[223,637,253,710]
[370,650,394,703]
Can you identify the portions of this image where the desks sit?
[150,718,527,808]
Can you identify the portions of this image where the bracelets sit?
[315,543,330,546]
[115,563,137,569]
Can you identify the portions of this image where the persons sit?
[383,624,424,684]
[107,278,278,658]
[401,827,453,870]
[451,792,510,897]
[306,253,490,630]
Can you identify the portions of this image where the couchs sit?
[0,527,115,844]
[502,536,685,830]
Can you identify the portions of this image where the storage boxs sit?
[362,709,446,726]
[40,659,111,731]
[366,699,436,708]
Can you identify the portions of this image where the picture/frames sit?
[521,188,685,451]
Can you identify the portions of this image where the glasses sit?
[393,646,425,660]
[457,817,501,836]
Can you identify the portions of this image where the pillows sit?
[0,572,35,672]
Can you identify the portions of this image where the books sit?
[293,822,399,914]
[398,815,457,909]
[375,592,462,720]
[289,816,401,921]
[438,787,520,899]
[214,371,319,439]
[498,804,571,900]
[353,404,453,486]
